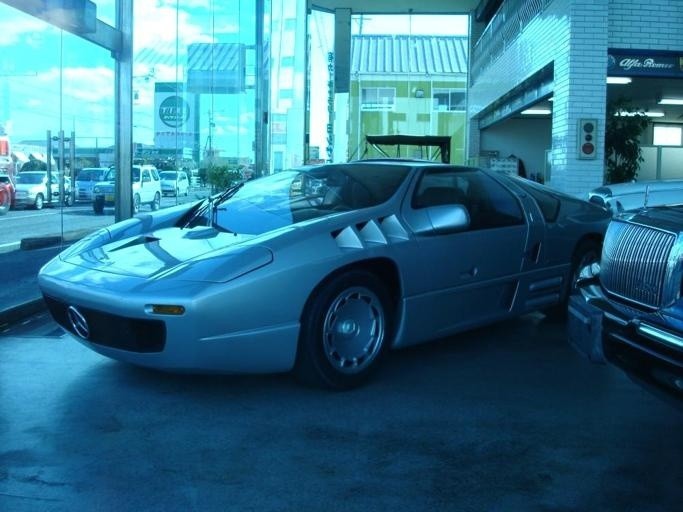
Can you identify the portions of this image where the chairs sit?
[327,183,470,234]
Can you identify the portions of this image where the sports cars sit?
[37,159,613,391]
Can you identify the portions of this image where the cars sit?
[0,136,199,215]
[568,178,683,399]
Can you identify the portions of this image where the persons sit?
[508,154,527,178]
[182,167,192,193]
